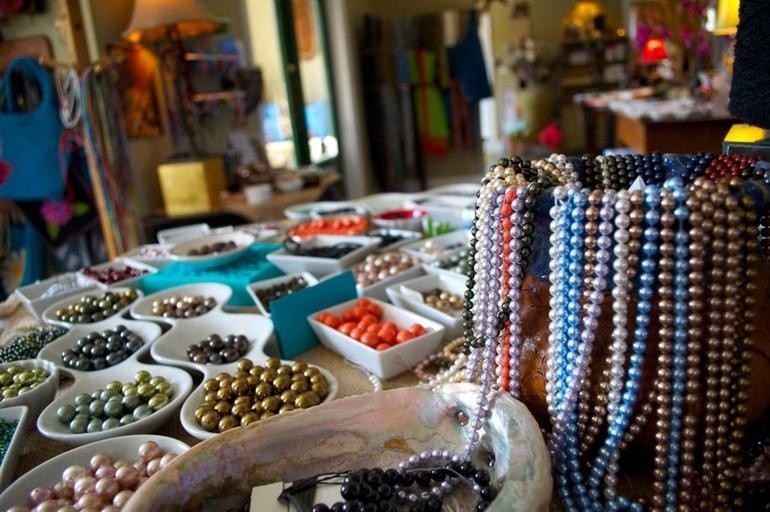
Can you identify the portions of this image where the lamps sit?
[120,0,231,161]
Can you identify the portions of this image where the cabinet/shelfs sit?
[558,30,629,154]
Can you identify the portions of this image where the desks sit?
[0,183,770,512]
[608,102,734,154]
[138,170,343,244]
[576,84,664,154]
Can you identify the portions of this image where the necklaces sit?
[311,150,770,512]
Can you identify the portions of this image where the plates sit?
[0,403,28,495]
[16,272,99,322]
[38,289,193,446]
[266,234,382,275]
[362,227,421,253]
[355,263,424,303]
[129,282,341,440]
[81,258,158,288]
[307,293,449,378]
[161,229,255,271]
[383,269,476,341]
[427,249,468,301]
[284,183,481,222]
[401,229,474,262]
[245,270,323,318]
[0,435,194,512]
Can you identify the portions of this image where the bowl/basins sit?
[371,208,427,231]
[120,382,551,512]
[0,359,59,423]
